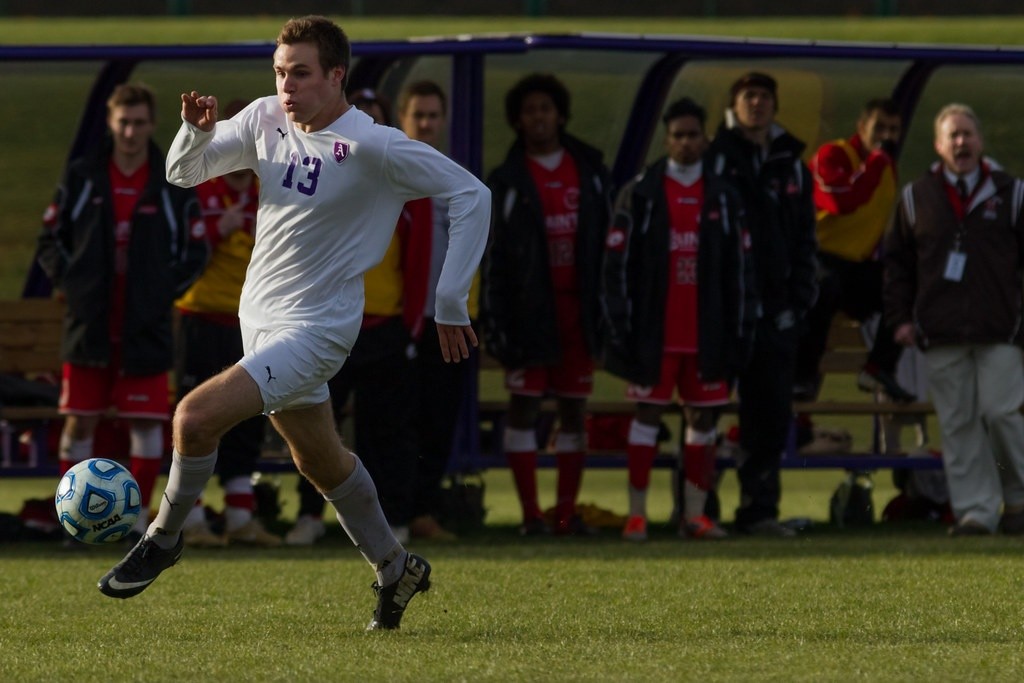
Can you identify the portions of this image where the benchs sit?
[0,395,942,528]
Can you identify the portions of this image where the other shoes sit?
[945,519,990,535]
[522,510,587,538]
[736,514,797,538]
[411,515,459,540]
[1003,511,1024,535]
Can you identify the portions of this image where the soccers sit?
[56,458,144,546]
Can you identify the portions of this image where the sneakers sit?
[856,369,918,407]
[622,515,648,541]
[679,513,729,539]
[388,524,410,544]
[364,552,432,632]
[800,426,853,458]
[224,521,285,546]
[284,514,326,545]
[183,524,225,547]
[99,530,186,599]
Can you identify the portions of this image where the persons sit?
[892,103,1024,535]
[285,87,412,547]
[96,14,492,629]
[19,72,918,547]
